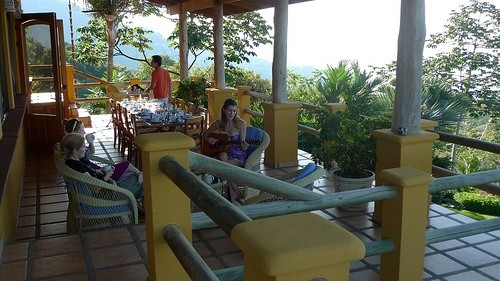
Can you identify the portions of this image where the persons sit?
[146,55,173,103]
[63,118,130,180]
[61,134,143,224]
[202,98,249,166]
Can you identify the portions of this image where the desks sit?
[116,99,201,151]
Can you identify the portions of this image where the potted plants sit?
[311,102,393,212]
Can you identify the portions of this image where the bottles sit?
[163,101,166,110]
[167,108,171,121]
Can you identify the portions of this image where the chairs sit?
[56,96,325,234]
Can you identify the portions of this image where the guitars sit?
[202,133,261,156]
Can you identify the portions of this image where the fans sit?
[81,0,142,21]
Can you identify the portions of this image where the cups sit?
[86,134,94,143]
[103,165,115,173]
[203,174,214,184]
[123,96,191,123]
